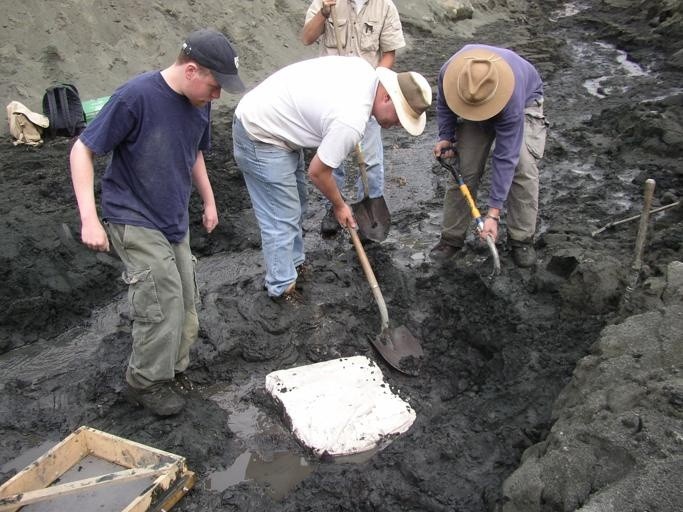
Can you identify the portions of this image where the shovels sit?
[346,220,424,375]
[331,5,391,241]
[437,146,523,297]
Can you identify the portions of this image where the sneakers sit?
[431,240,462,260]
[511,246,538,267]
[321,208,370,244]
[271,263,313,311]
[126,373,201,416]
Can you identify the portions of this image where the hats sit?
[443,49,515,122]
[181,30,246,94]
[376,66,432,137]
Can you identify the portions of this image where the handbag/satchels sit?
[6,101,50,147]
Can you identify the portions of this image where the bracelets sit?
[486,212,501,222]
[320,8,328,18]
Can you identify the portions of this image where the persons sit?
[429,44,545,268]
[69,30,245,415]
[233,56,433,325]
[302,0,406,236]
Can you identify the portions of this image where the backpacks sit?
[42,83,87,139]
